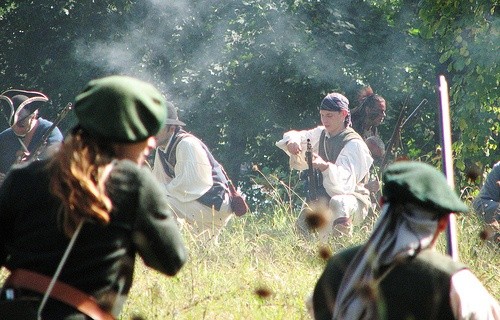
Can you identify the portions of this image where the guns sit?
[438,75,459,257]
[384,98,428,149]
[305,138,317,201]
[25,101,72,163]
[377,94,411,173]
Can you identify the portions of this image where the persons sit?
[349,83,386,171]
[0,89,64,185]
[474,161,500,225]
[276,92,373,241]
[0,75,188,320]
[152,102,232,236]
[306,160,500,320]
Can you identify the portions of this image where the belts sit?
[8,270,116,320]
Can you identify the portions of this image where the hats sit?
[73,74,168,144]
[0,89,49,129]
[165,101,186,126]
[381,159,468,216]
